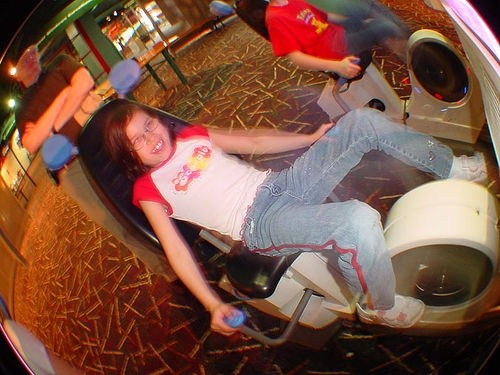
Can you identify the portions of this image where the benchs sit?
[180,14,224,44]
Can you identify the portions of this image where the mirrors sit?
[0,0,500,375]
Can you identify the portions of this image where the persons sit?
[13,41,210,305]
[264,1,414,80]
[103,101,490,336]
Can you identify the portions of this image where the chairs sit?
[233,0,373,81]
[77,97,303,299]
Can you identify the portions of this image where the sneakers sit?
[356,294,425,328]
[448,152,487,182]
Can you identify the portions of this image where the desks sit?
[101,35,187,102]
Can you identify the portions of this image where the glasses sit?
[129,118,159,153]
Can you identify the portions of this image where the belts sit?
[54,155,76,175]
